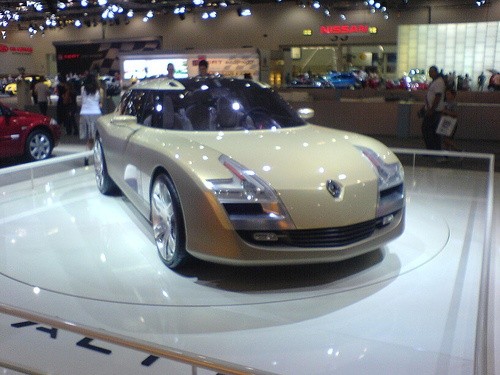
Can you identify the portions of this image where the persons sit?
[45,72,80,91]
[198,60,210,77]
[64,73,81,136]
[440,68,500,92]
[30,73,52,115]
[79,73,102,147]
[166,63,175,78]
[56,74,66,127]
[421,66,447,150]
[95,71,136,91]
[0,67,25,94]
[440,88,463,151]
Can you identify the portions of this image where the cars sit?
[313,72,361,89]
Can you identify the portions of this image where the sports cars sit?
[5,76,52,96]
[92,76,407,271]
[0,101,61,161]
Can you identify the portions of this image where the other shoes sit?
[436,158,448,163]
[459,149,465,160]
